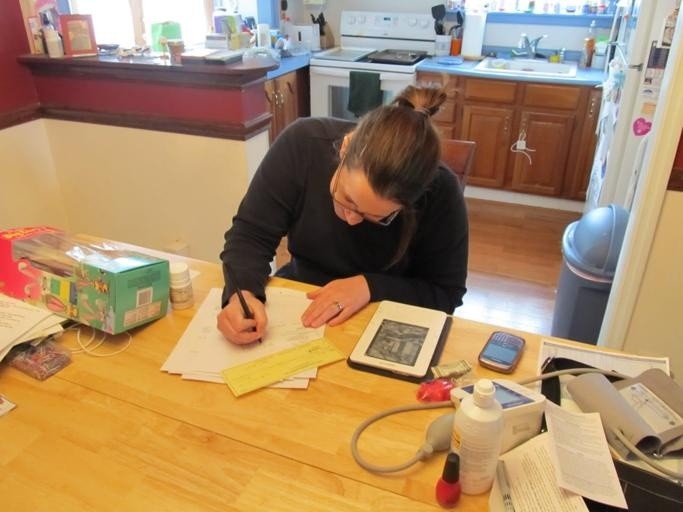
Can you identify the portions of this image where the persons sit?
[216,70,468,344]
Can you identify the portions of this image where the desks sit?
[0,232,670,512]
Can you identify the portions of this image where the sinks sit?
[474,55,579,77]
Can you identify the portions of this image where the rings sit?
[335,300,341,310]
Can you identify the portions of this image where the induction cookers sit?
[367,48,426,64]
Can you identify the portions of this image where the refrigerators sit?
[585,0,657,212]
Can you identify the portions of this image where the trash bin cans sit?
[552,204,630,345]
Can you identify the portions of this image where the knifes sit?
[311,13,327,37]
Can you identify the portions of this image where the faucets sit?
[520,33,549,59]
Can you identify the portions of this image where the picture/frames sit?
[60,15,98,55]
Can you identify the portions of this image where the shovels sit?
[432,5,445,24]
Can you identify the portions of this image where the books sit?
[178,44,242,64]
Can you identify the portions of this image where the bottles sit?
[166,263,194,311]
[660,8,680,47]
[491,0,618,15]
[434,452,460,509]
[556,48,567,61]
[38,6,64,58]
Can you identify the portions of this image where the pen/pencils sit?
[225,262,262,343]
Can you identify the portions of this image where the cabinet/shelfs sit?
[416,70,603,202]
[263,73,299,153]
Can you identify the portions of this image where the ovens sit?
[309,66,416,123]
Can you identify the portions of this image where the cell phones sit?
[478,331,525,374]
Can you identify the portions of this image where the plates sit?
[96,44,119,51]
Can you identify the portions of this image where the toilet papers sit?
[461,12,487,57]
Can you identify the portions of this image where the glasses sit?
[331,154,398,227]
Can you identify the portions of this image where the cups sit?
[257,24,271,49]
[592,53,605,70]
[436,34,452,58]
[451,38,461,56]
[167,38,183,58]
